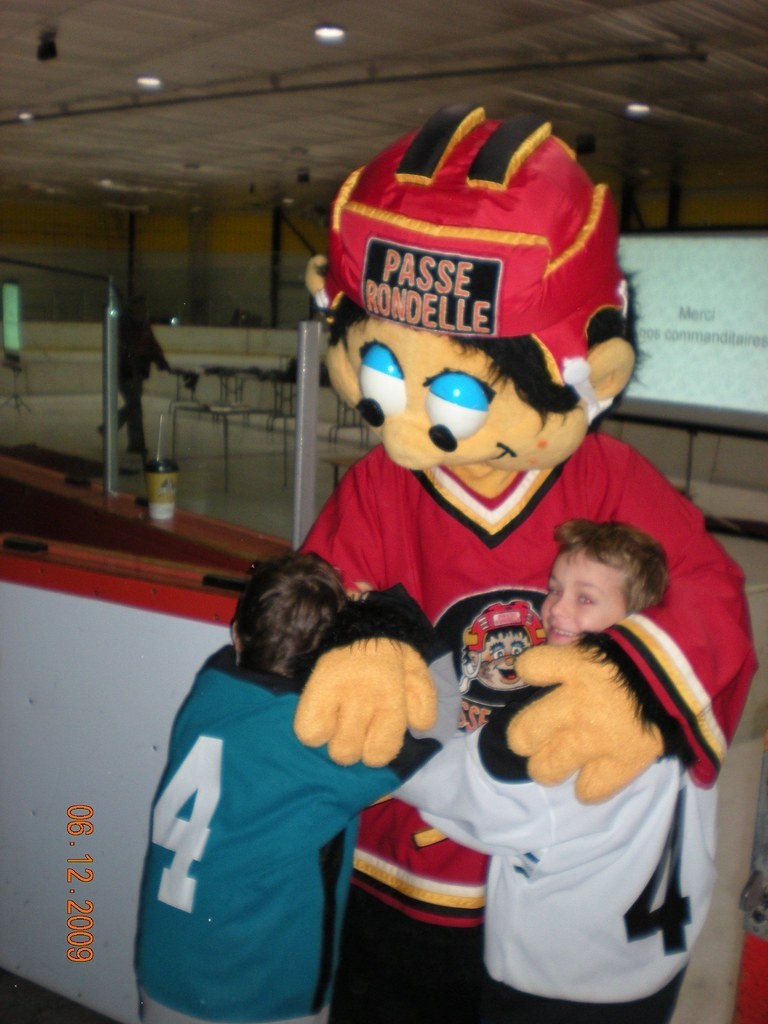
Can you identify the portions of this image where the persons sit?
[390,514,719,1023]
[98,296,174,471]
[133,553,460,1023]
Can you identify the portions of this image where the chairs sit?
[178,356,369,447]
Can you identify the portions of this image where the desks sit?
[320,453,363,491]
[174,402,290,496]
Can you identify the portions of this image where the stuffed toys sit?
[291,100,760,1023]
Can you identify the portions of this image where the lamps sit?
[37,40,57,60]
[575,134,594,155]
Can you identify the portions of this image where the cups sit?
[145,460,180,520]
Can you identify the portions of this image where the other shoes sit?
[127,445,148,452]
[99,425,104,436]
